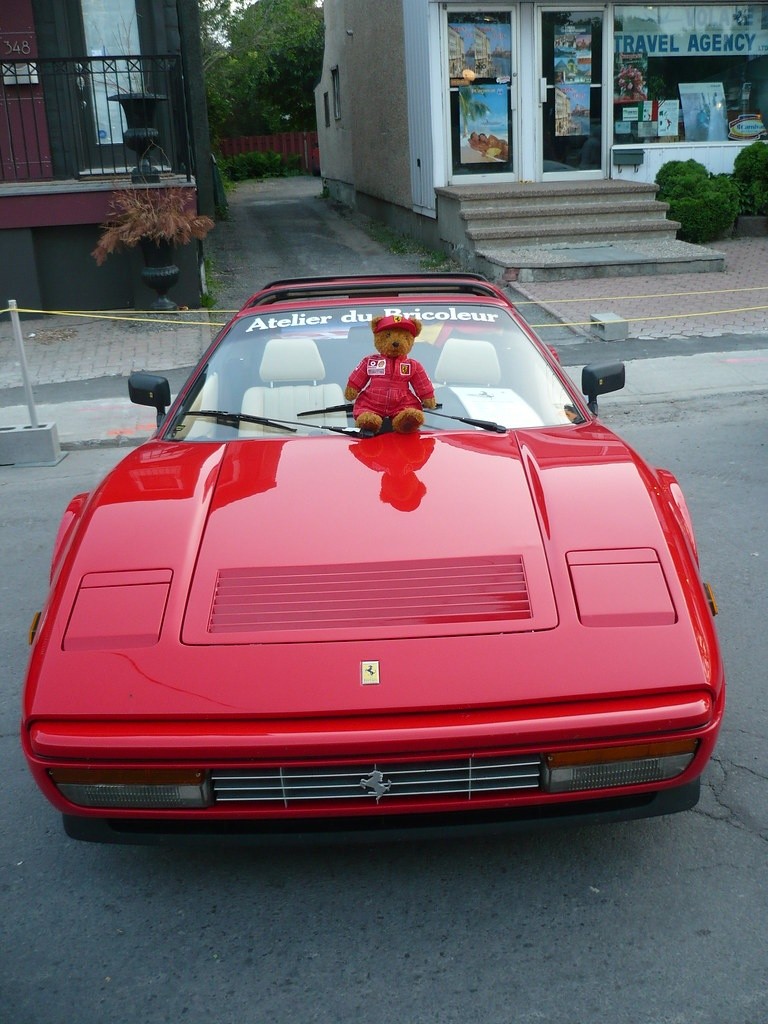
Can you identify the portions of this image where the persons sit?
[469,132,509,161]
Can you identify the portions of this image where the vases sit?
[139,234,170,269]
[108,93,171,128]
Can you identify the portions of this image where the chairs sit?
[238,338,348,434]
[313,327,440,385]
[434,338,543,429]
[465,142,507,162]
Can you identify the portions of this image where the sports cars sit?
[19,272,727,838]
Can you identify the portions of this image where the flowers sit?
[90,167,216,268]
[619,65,647,99]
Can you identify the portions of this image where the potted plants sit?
[654,141,768,244]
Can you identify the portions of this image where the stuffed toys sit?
[345,315,435,432]
